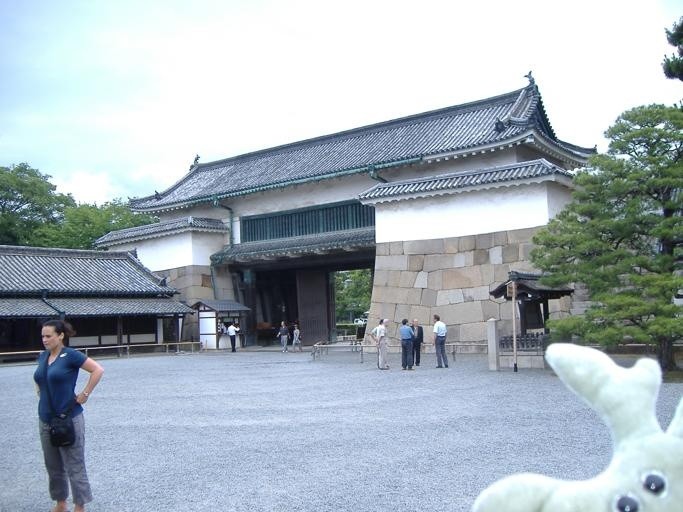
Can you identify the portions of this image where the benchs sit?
[0,341,203,358]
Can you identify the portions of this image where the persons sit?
[291,323,302,353]
[370,319,390,367]
[33,319,105,512]
[409,319,423,366]
[228,322,240,353]
[431,314,449,368]
[399,319,416,371]
[277,320,290,352]
[376,318,390,370]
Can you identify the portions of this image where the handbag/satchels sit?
[299,335,303,341]
[46,413,76,448]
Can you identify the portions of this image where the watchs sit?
[84,391,89,397]
